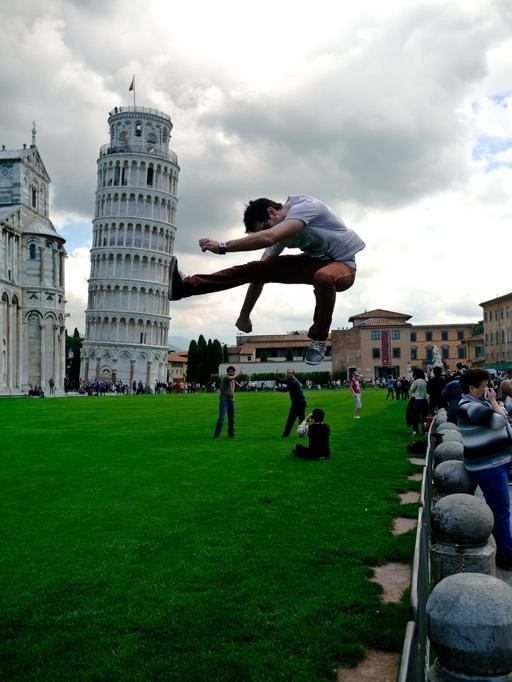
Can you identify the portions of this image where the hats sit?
[352,370,360,376]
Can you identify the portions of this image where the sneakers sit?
[301,339,329,366]
[167,255,187,303]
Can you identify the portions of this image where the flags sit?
[129,81,134,91]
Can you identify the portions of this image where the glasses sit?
[262,219,272,230]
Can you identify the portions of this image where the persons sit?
[166,193,366,366]
[23,373,386,401]
[292,408,330,460]
[349,371,362,419]
[274,368,307,437]
[213,367,251,441]
[455,368,511,571]
[387,360,511,491]
